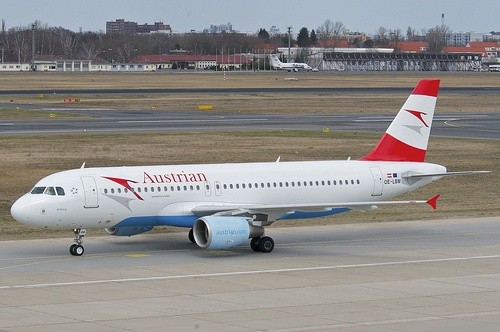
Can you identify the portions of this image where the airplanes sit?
[269,55,312,73]
[9,78,493,256]
